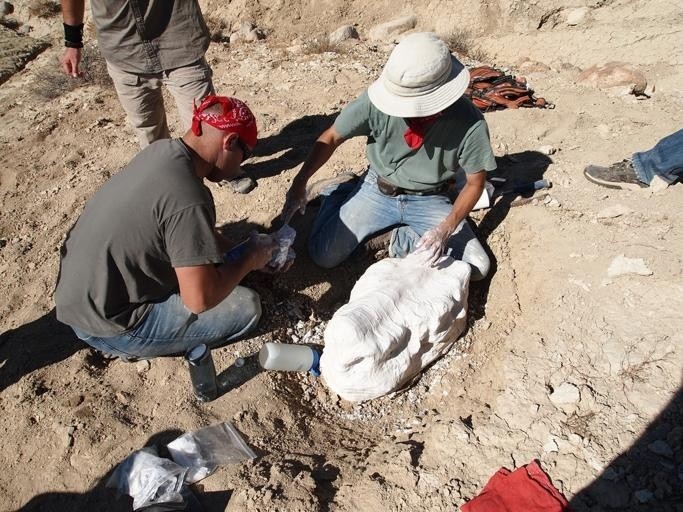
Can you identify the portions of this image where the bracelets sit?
[62,23,83,42]
[64,41,83,48]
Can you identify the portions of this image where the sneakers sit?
[228,171,254,194]
[360,228,396,251]
[583,158,649,192]
[307,172,357,205]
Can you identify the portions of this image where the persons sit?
[56,1,254,193]
[50,93,284,358]
[583,130,682,195]
[283,32,494,284]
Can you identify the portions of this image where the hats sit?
[368,32,471,117]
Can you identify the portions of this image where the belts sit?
[376,178,448,197]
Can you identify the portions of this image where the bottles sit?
[258,342,320,379]
[221,236,254,265]
[186,343,218,403]
